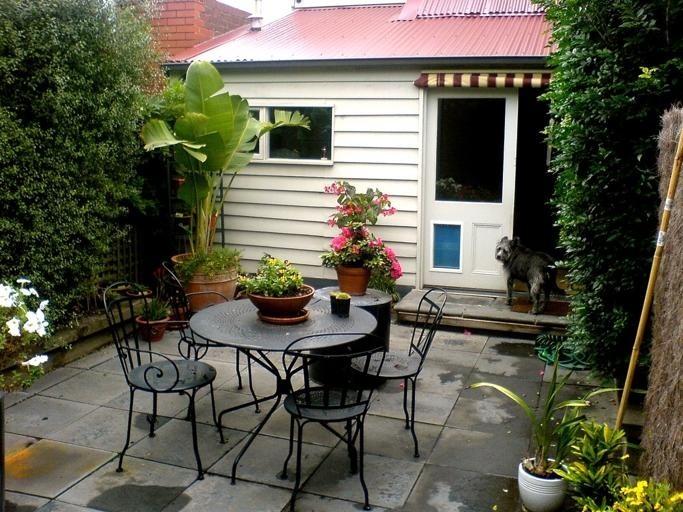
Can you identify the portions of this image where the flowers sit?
[316,175,404,279]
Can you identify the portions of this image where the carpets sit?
[511,296,569,316]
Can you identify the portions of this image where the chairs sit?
[88,277,227,484]
[158,261,265,432]
[272,328,389,510]
[338,282,450,460]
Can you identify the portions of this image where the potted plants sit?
[234,252,314,313]
[335,293,351,318]
[134,294,169,342]
[465,338,648,512]
[139,60,309,312]
[329,291,340,315]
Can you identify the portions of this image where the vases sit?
[332,267,370,298]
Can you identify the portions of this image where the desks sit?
[186,295,381,487]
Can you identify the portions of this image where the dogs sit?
[494,235,568,315]
